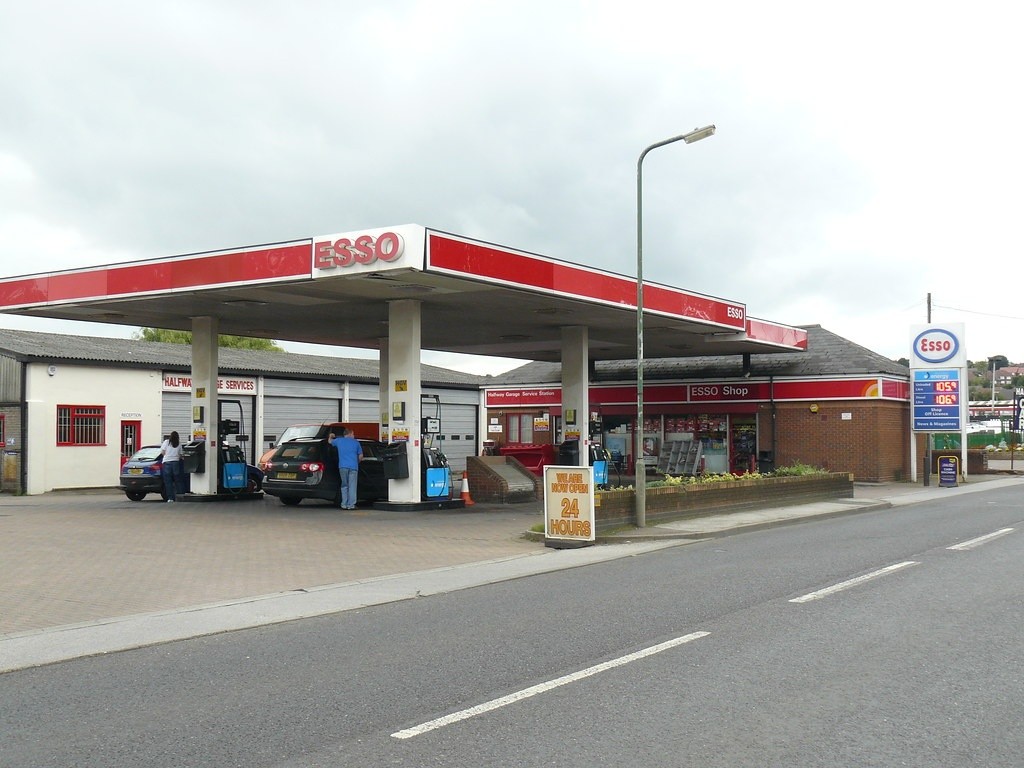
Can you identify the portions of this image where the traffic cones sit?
[458,471,475,505]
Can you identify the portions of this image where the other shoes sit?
[341,503,347,509]
[168,499,174,503]
[347,504,355,509]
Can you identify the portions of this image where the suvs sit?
[120,444,265,500]
[261,436,388,506]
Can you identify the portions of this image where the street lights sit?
[637,125,715,528]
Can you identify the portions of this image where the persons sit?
[328,429,364,510]
[161,431,182,503]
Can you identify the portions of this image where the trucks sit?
[258,423,379,472]
